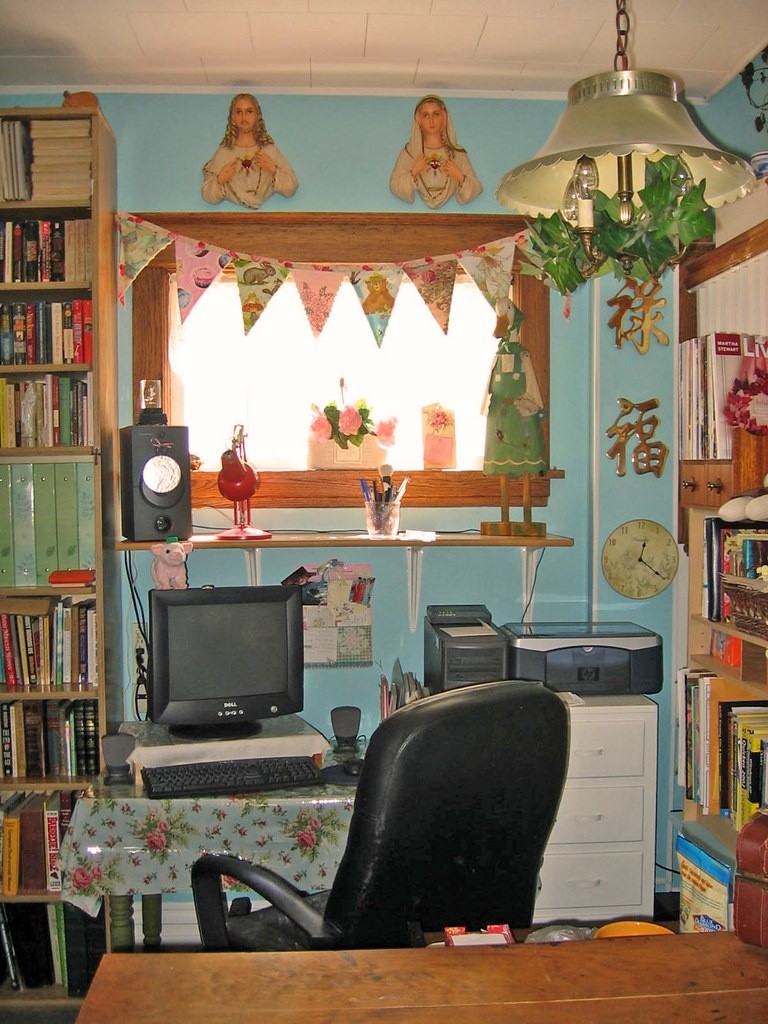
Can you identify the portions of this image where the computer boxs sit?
[422,615,512,694]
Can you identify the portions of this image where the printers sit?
[499,622,663,695]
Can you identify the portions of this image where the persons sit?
[390,94,483,208]
[351,577,376,605]
[286,573,309,586]
[202,93,298,209]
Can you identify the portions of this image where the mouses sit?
[343,756,365,777]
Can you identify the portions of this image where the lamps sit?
[217,423,274,540]
[495,0,757,275]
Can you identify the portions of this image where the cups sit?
[364,502,402,540]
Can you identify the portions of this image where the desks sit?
[56,738,368,954]
[77,929,768,1024]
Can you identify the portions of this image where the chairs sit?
[191,682,568,954]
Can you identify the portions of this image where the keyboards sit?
[140,755,325,801]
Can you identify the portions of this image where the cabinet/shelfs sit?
[0,103,127,1015]
[531,694,659,924]
[679,216,768,829]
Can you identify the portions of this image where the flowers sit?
[307,378,399,450]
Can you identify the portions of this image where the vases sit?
[304,434,389,470]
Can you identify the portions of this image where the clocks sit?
[601,519,679,600]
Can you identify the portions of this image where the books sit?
[0,700,99,777]
[49,569,96,588]
[0,372,94,447]
[0,300,91,365]
[701,516,768,623]
[0,789,83,896]
[0,901,106,997]
[381,673,430,720]
[0,118,92,201]
[0,219,92,283]
[0,596,98,686]
[685,670,768,831]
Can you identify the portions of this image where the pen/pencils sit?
[361,477,411,529]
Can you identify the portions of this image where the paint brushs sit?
[378,463,393,503]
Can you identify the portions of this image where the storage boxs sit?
[733,804,768,948]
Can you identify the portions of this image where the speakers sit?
[100,733,135,786]
[330,705,361,753]
[119,425,194,541]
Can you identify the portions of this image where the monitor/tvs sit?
[146,589,304,741]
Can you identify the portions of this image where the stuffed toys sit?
[63,91,98,106]
[718,474,768,522]
[151,537,193,590]
